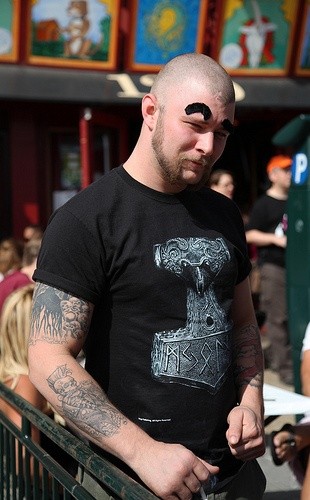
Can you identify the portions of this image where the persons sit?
[25,52,267,500]
[0,218,75,476]
[209,156,310,500]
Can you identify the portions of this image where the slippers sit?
[270,423,298,467]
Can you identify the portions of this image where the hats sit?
[268,156,292,172]
